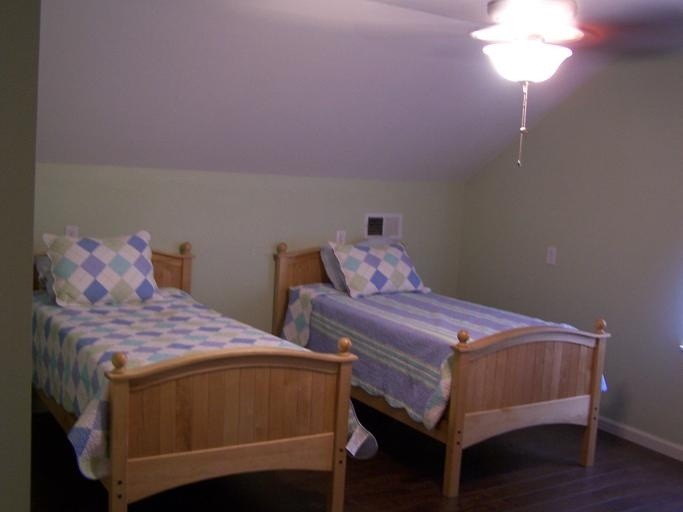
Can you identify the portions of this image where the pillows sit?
[43,230,159,309]
[36,254,55,301]
[328,238,431,299]
[320,245,346,290]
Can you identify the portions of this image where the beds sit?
[271,242,612,498]
[34,241,359,511]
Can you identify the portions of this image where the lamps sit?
[469,0,584,168]
